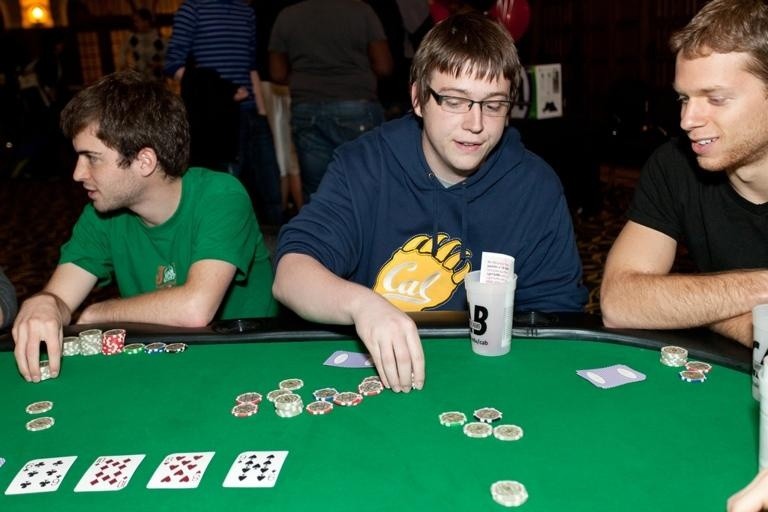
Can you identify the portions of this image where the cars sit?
[751,302,768,404]
[757,367,768,472]
[463,270,519,358]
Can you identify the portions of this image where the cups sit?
[427,84,515,118]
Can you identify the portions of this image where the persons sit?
[727,470,768,512]
[164,0,432,232]
[598,0,768,350]
[0,265,19,331]
[272,12,591,394]
[12,68,278,384]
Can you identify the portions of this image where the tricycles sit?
[5,455,78,495]
[222,451,289,488]
[146,451,216,489]
[576,364,646,389]
[534,64,563,120]
[322,351,375,368]
[73,454,146,492]
